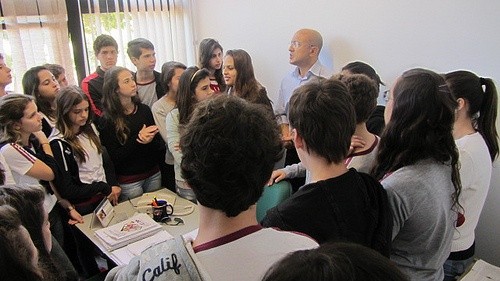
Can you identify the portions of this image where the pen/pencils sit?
[152,198,166,206]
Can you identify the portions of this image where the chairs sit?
[255,180,292,225]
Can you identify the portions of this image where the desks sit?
[73,187,201,267]
[457,256,500,281]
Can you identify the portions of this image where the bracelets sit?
[67,205,75,211]
[41,142,49,146]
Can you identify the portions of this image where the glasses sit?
[291,41,313,48]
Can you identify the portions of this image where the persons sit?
[0,28,500,281]
[105,93,320,281]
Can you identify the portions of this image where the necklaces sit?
[467,130,477,135]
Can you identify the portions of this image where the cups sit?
[151,199,174,222]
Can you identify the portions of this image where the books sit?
[94,211,174,268]
